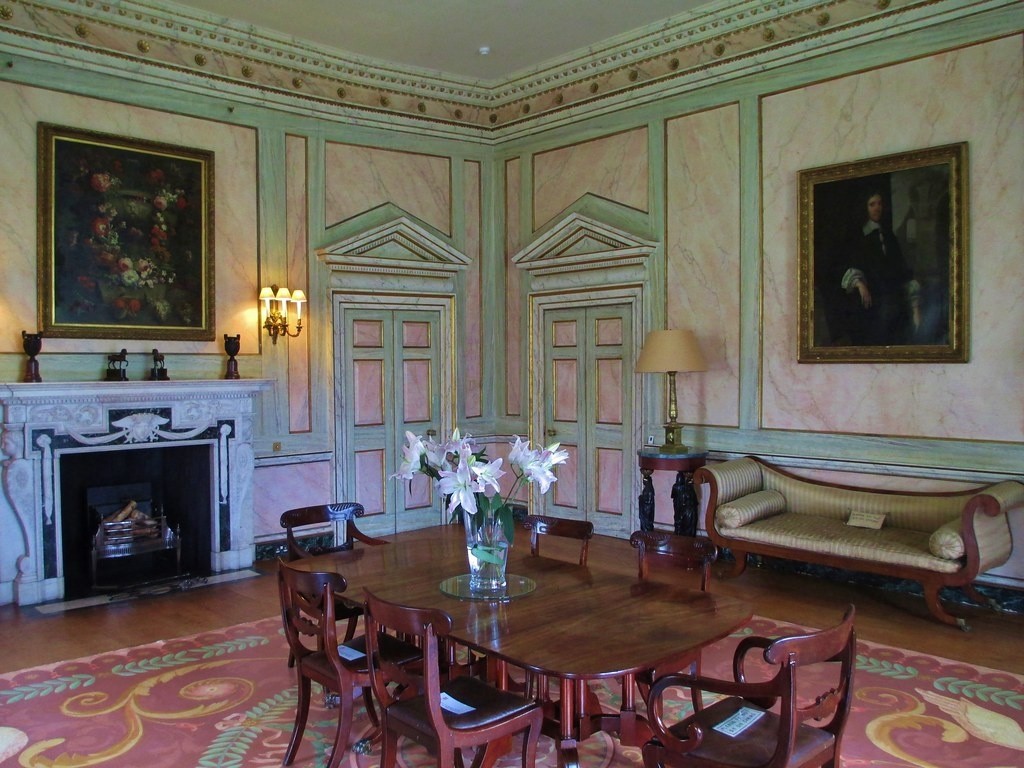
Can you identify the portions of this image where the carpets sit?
[1,606,1024,768]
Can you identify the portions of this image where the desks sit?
[282,535,755,768]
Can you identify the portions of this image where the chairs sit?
[279,502,394,670]
[360,585,545,768]
[276,557,425,768]
[480,513,596,722]
[640,602,861,768]
[615,530,720,726]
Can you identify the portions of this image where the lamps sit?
[258,286,308,345]
[634,328,712,455]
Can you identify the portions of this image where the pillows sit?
[929,514,967,560]
[714,487,788,530]
[847,510,886,529]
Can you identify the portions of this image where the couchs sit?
[693,454,1024,631]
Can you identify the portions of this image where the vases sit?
[459,503,515,593]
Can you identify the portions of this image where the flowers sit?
[387,427,572,594]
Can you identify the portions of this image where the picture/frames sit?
[36,120,218,344]
[795,140,971,364]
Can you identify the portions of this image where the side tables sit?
[626,447,710,541]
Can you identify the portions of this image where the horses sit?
[108,349,128,369]
[152,348,164,368]
